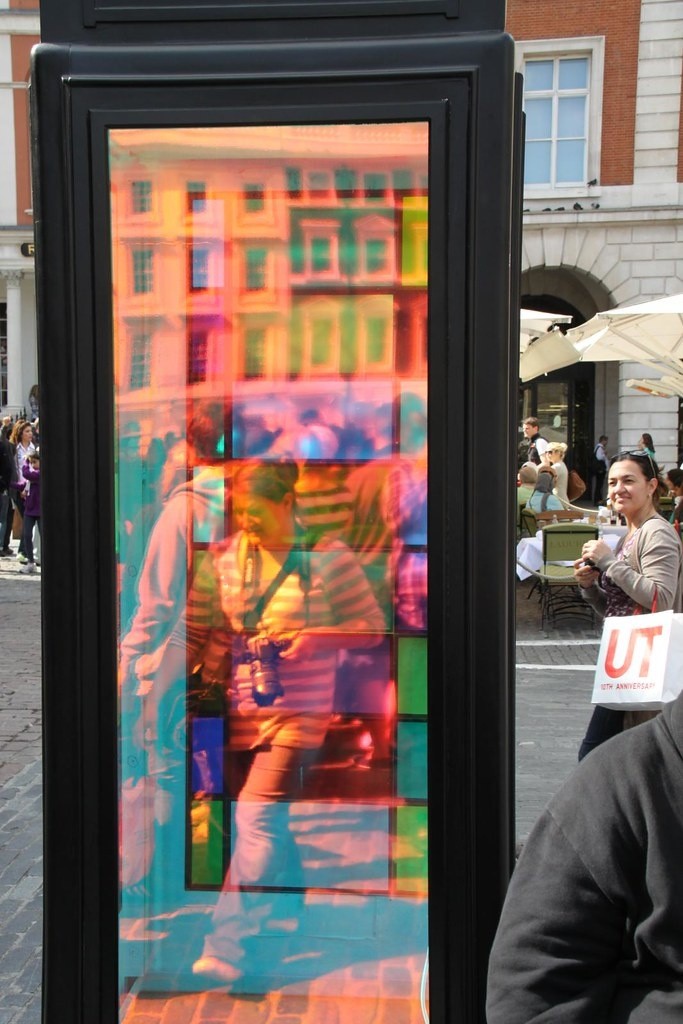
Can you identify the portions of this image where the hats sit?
[539,467,559,485]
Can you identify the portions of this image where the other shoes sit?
[18,562,38,574]
[1,548,14,558]
[15,554,29,563]
[190,954,249,984]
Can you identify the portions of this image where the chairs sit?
[516,494,683,635]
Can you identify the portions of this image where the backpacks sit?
[590,447,606,477]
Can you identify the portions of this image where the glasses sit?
[544,448,555,454]
[612,447,659,479]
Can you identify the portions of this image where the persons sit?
[572,451,683,763]
[518,417,585,540]
[486,691,683,1024]
[594,436,610,506]
[638,434,683,511]
[120,398,429,985]
[0,415,41,574]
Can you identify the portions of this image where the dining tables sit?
[518,534,622,603]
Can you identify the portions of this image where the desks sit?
[594,526,629,538]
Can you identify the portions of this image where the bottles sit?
[551,513,558,524]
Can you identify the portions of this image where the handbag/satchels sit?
[156,672,232,781]
[565,470,587,502]
[590,580,683,712]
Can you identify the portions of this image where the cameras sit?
[243,639,292,707]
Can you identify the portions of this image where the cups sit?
[600,509,611,526]
[610,511,617,526]
[606,498,612,510]
[618,512,626,526]
[588,512,598,524]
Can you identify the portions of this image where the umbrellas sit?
[566,293,683,383]
[520,307,572,353]
